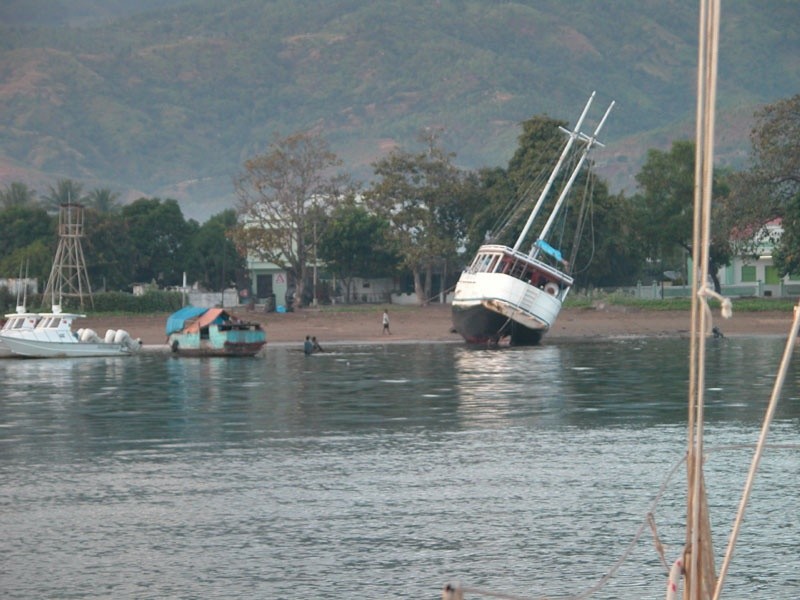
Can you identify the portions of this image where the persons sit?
[305,335,324,354]
[382,310,392,335]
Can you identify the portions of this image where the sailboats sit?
[445,88,618,350]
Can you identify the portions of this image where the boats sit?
[0,257,144,359]
[165,306,269,358]
